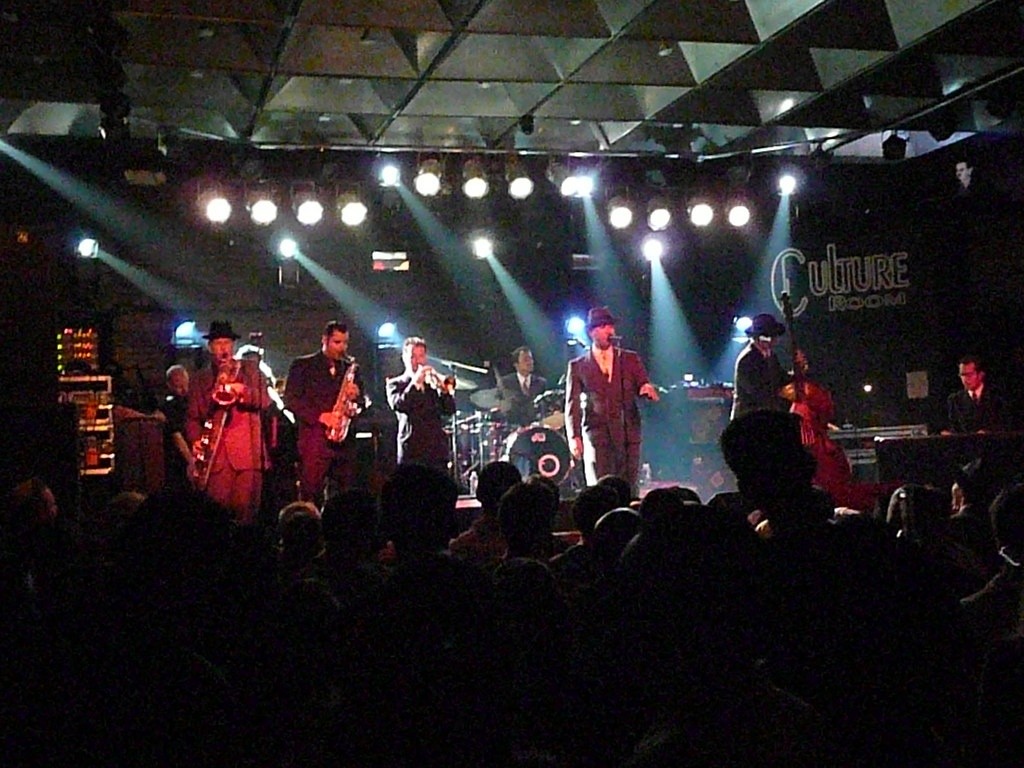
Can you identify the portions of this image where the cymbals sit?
[468,388,521,410]
[439,374,480,390]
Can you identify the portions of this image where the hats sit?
[583,307,622,328]
[277,500,324,527]
[202,319,240,340]
[745,314,786,338]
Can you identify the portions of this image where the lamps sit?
[331,176,369,226]
[879,130,908,161]
[602,184,637,230]
[369,152,404,189]
[644,184,676,233]
[242,172,283,228]
[723,188,754,226]
[682,184,715,226]
[544,155,600,197]
[503,153,534,200]
[461,154,492,197]
[286,178,326,226]
[194,177,232,226]
[413,152,453,196]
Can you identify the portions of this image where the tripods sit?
[457,404,503,480]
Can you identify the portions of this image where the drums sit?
[533,389,569,429]
[507,425,572,487]
[449,422,513,463]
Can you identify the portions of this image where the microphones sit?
[606,334,623,342]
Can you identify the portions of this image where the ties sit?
[524,380,528,395]
[973,394,977,406]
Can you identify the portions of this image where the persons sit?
[789,349,853,510]
[385,336,457,471]
[565,307,658,497]
[730,314,809,420]
[941,353,1012,500]
[0,462,1024,768]
[494,347,551,481]
[101,319,367,526]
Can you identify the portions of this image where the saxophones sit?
[324,351,366,440]
[187,354,235,491]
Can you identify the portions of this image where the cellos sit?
[778,293,854,497]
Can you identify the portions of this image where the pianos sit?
[877,433,1024,474]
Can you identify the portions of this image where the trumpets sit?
[422,363,457,395]
